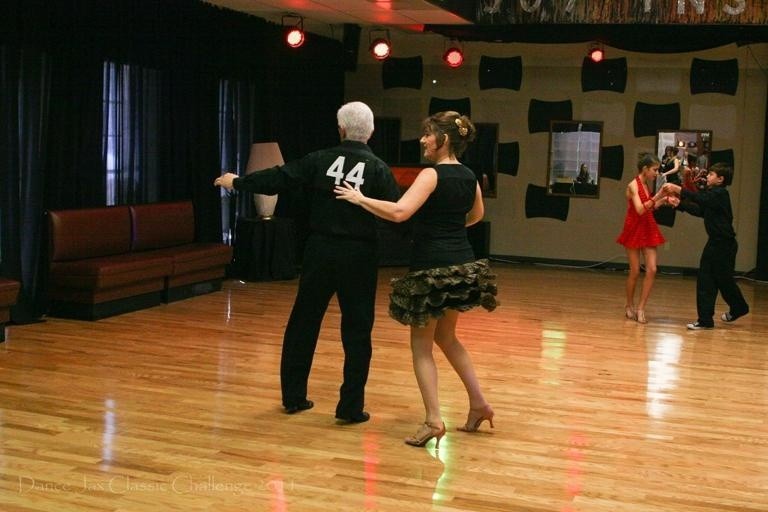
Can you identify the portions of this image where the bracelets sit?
[666,194,669,203]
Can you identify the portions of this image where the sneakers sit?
[687,321,714,329]
[721,312,734,322]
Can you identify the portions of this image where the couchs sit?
[130,201,234,304]
[39,207,173,321]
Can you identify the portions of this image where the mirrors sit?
[654,131,712,195]
[366,117,402,166]
[547,121,604,198]
[462,122,500,199]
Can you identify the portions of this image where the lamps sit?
[589,43,605,62]
[441,38,464,68]
[244,143,286,220]
[367,29,392,61]
[280,14,306,48]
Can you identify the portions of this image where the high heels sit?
[625,304,647,324]
[457,405,494,433]
[405,422,445,449]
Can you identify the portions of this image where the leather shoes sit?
[335,411,369,421]
[284,401,312,412]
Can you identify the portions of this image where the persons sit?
[662,162,750,332]
[213,99,402,425]
[659,144,708,194]
[616,150,683,324]
[333,111,499,449]
[576,162,594,184]
